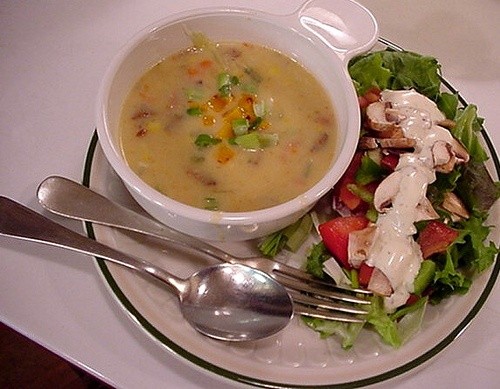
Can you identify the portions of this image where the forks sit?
[36,174,374,325]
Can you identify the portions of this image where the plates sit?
[81,40,499,389]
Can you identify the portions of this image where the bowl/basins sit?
[92,0,379,245]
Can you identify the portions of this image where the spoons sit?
[0,192,295,341]
